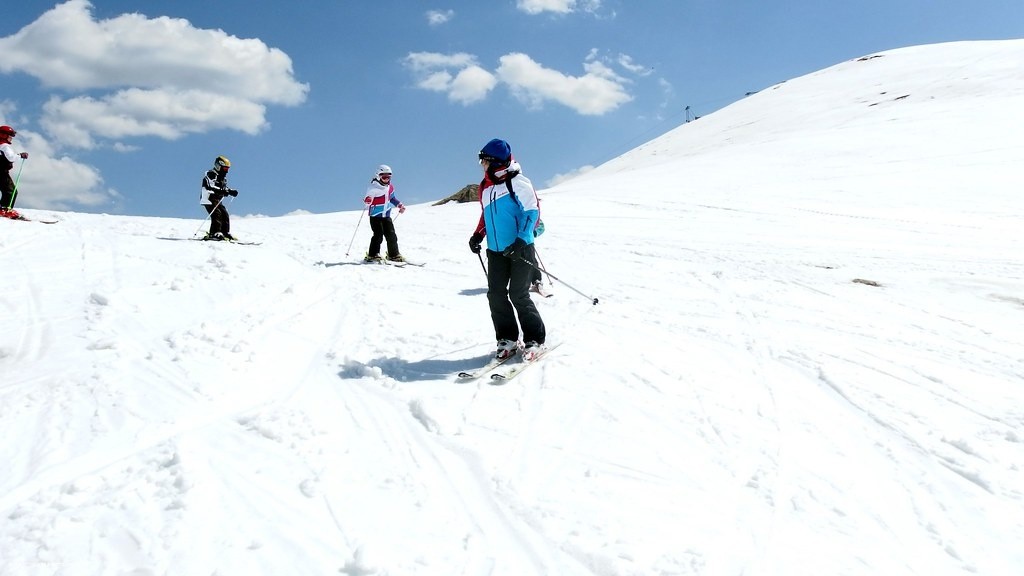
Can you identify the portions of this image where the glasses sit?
[223,166,229,171]
[478,157,486,165]
[380,175,392,180]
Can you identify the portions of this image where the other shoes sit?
[0,205,19,217]
[208,232,225,241]
[223,232,234,240]
[531,278,542,289]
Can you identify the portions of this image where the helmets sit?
[0,125,16,144]
[213,156,231,176]
[376,165,393,181]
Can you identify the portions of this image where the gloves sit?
[21,153,28,159]
[397,203,405,213]
[365,196,372,204]
[505,236,527,263]
[222,189,229,197]
[228,189,238,197]
[469,232,485,253]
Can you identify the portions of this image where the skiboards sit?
[363,255,427,268]
[158,235,263,246]
[527,285,554,298]
[4,212,59,224]
[457,339,563,381]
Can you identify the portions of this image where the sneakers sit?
[495,338,522,358]
[363,252,383,262]
[521,339,545,362]
[384,252,407,265]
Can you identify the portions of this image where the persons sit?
[0,126,29,218]
[468,138,546,363]
[363,165,407,262]
[508,160,540,289]
[200,156,239,241]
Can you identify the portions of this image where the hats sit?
[480,139,511,167]
